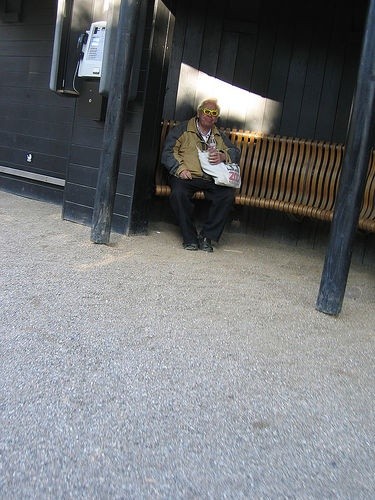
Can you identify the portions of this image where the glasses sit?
[200,108,219,116]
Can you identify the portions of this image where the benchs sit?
[156,120,375,232]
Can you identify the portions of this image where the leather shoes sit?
[184,241,198,250]
[198,235,213,251]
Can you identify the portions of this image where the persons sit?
[158,97,244,252]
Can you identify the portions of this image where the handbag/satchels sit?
[196,147,243,188]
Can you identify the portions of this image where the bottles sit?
[207,133,216,152]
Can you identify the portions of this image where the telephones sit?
[76,21,107,77]
